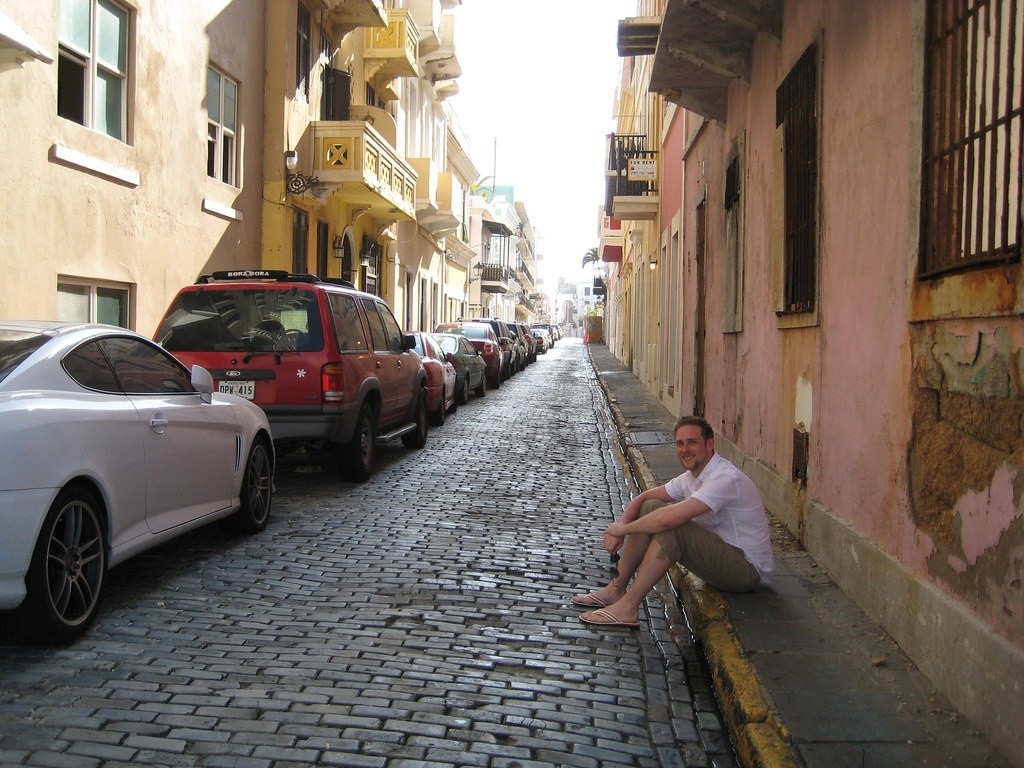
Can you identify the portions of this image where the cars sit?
[397,331,457,426]
[431,332,489,405]
[0,319,275,645]
[454,316,563,380]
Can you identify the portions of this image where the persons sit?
[571,417,772,629]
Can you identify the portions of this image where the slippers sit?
[579,608,640,628]
[571,593,610,607]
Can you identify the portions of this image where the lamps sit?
[469,262,484,284]
[286,151,298,169]
[360,250,370,267]
[597,296,605,306]
[650,260,657,269]
[332,234,344,259]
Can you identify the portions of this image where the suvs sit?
[148,268,427,483]
[434,321,510,389]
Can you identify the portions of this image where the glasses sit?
[610,552,620,586]
